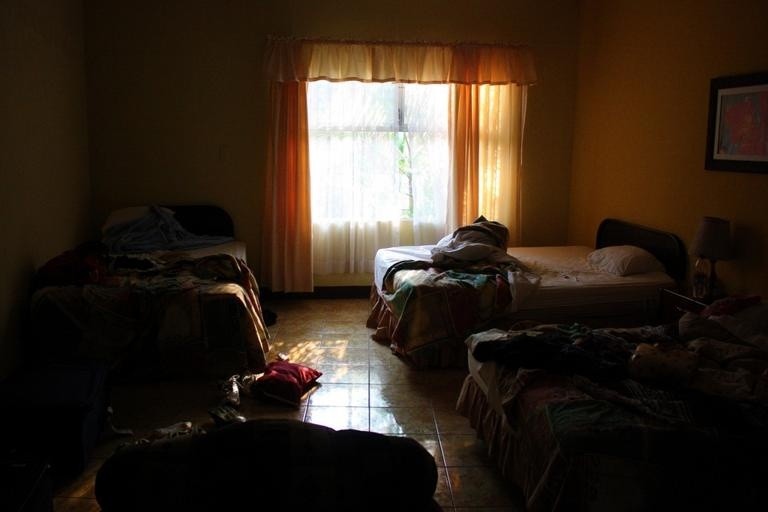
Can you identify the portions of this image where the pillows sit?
[586,245,658,279]
[104,205,176,230]
[708,303,768,352]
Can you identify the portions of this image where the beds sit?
[374,217,689,371]
[467,303,768,512]
[31,203,247,384]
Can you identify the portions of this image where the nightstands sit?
[657,280,737,328]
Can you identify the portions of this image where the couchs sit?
[94,418,444,512]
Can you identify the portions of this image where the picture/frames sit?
[703,70,768,174]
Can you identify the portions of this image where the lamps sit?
[688,215,734,298]
